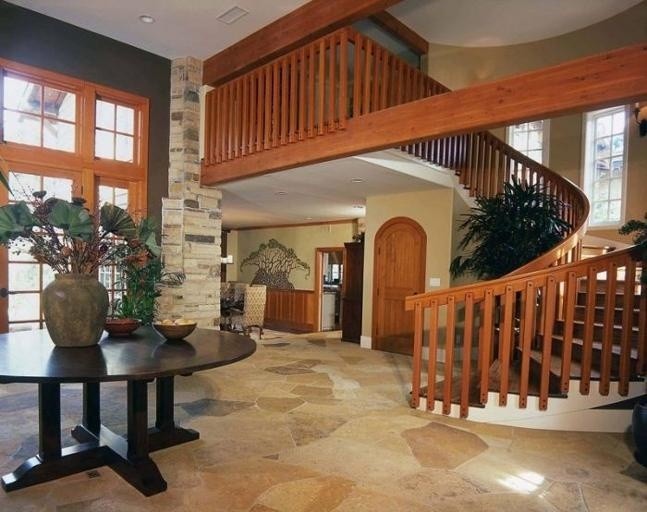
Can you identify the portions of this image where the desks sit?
[0,326,256,497]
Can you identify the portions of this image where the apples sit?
[154,317,197,325]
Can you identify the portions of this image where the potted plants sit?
[449,175,575,361]
[0,190,160,347]
[618,213,647,468]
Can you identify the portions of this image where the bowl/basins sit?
[103,318,143,337]
[151,318,197,342]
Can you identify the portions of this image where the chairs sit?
[220,282,267,340]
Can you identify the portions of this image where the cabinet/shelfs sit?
[323,292,336,331]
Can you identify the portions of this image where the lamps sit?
[635,102,647,137]
